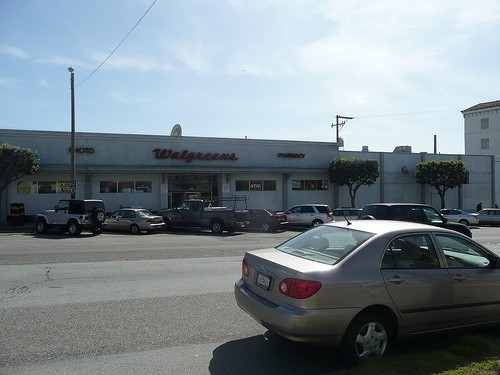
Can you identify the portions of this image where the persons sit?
[495,204,498,208]
[476,202,483,212]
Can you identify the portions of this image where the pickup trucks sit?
[156,199,250,235]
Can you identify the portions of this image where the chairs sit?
[311,236,328,251]
[402,239,420,258]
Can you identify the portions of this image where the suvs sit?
[352,203,472,253]
[35,199,106,237]
[275,204,336,227]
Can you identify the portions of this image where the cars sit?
[246,208,289,233]
[234,219,500,368]
[104,208,166,235]
[333,207,363,221]
[468,208,500,227]
[439,208,479,226]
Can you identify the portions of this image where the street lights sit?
[67,67,76,200]
